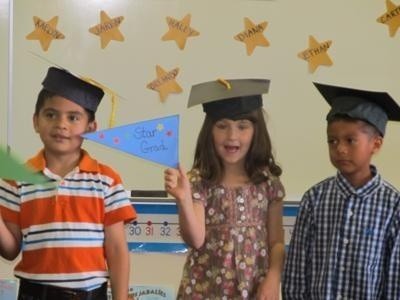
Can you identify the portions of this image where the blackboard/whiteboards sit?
[8,1,400,207]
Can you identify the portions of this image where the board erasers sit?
[130,190,168,198]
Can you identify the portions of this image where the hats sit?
[187,79,270,118]
[28,50,127,129]
[312,81,399,136]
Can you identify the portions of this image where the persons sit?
[164,78,285,300]
[0,67,138,300]
[281,82,400,300]
